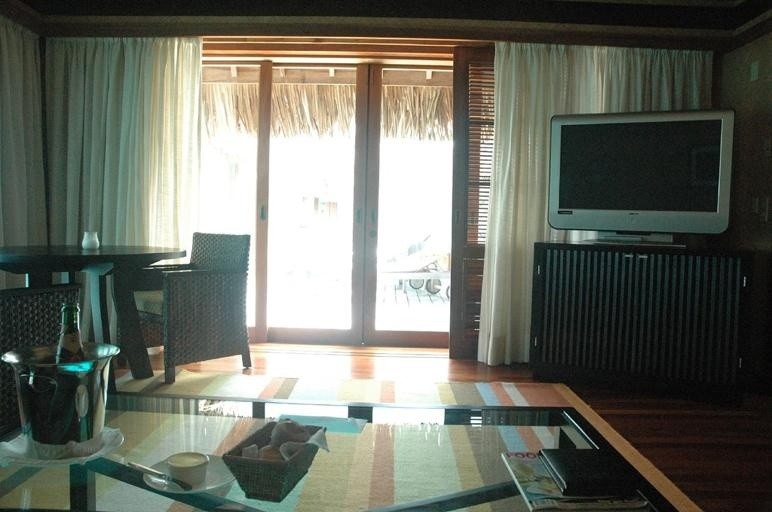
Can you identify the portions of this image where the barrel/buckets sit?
[0,342,121,459]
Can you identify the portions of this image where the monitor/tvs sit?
[547,108,734,247]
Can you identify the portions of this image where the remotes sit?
[596,235,644,243]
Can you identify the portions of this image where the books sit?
[501,447,650,511]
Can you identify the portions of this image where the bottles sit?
[55,302,88,357]
[83,231,99,250]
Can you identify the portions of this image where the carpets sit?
[0,369,706,512]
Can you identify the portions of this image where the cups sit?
[165,451,209,482]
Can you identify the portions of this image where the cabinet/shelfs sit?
[530,241,754,407]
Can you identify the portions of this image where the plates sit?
[138,455,236,492]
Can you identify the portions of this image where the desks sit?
[0,394,680,512]
[0,246,186,380]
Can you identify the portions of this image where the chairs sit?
[112,232,251,386]
[0,283,83,439]
